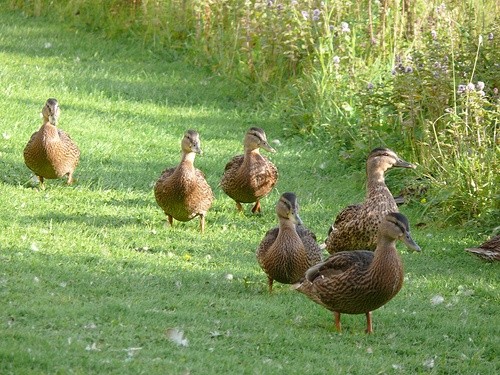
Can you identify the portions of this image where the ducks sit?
[153,129,216,234]
[464,231,500,263]
[220,126,278,220]
[23,98,82,191]
[295,212,423,335]
[319,146,417,256]
[254,193,323,296]
